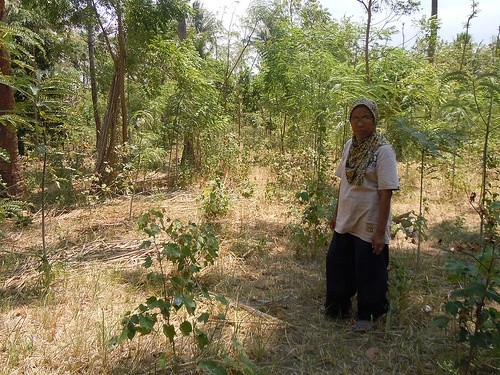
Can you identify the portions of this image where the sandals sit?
[352,321,374,334]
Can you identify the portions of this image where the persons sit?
[325,99,399,332]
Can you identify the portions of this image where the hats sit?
[348,97,378,124]
